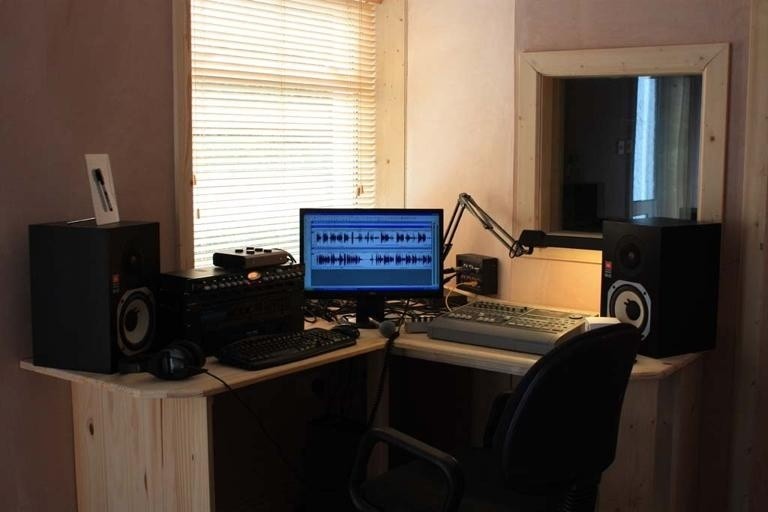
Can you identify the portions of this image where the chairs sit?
[347,321,640,511]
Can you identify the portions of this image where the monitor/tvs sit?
[298,206,441,327]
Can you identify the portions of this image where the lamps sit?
[444,191,549,269]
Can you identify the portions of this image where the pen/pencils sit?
[95,168,113,211]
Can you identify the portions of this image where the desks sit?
[22,300,720,511]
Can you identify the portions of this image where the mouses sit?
[330,323,361,338]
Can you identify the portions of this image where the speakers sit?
[29,219,161,376]
[598,213,722,359]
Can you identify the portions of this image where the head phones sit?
[142,339,207,377]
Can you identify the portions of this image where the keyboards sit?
[215,323,356,371]
